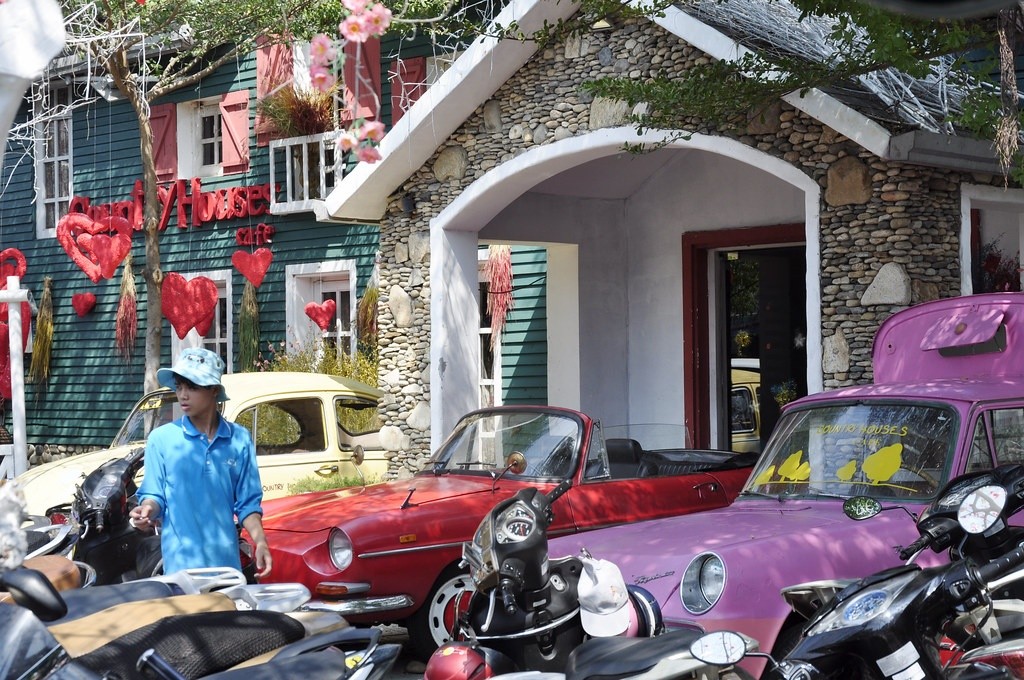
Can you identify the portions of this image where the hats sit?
[576,556,629,637]
[155,348,231,403]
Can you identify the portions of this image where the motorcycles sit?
[411,454,759,679]
[763,467,1024,679]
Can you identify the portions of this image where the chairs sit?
[525,436,573,476]
[587,438,658,479]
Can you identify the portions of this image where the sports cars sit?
[234,404,765,662]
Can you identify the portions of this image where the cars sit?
[544,294,1023,680]
[0,372,390,565]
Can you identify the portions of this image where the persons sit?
[128,348,272,591]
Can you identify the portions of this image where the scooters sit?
[0,555,411,680]
[19,447,262,593]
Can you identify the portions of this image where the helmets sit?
[424,644,494,680]
